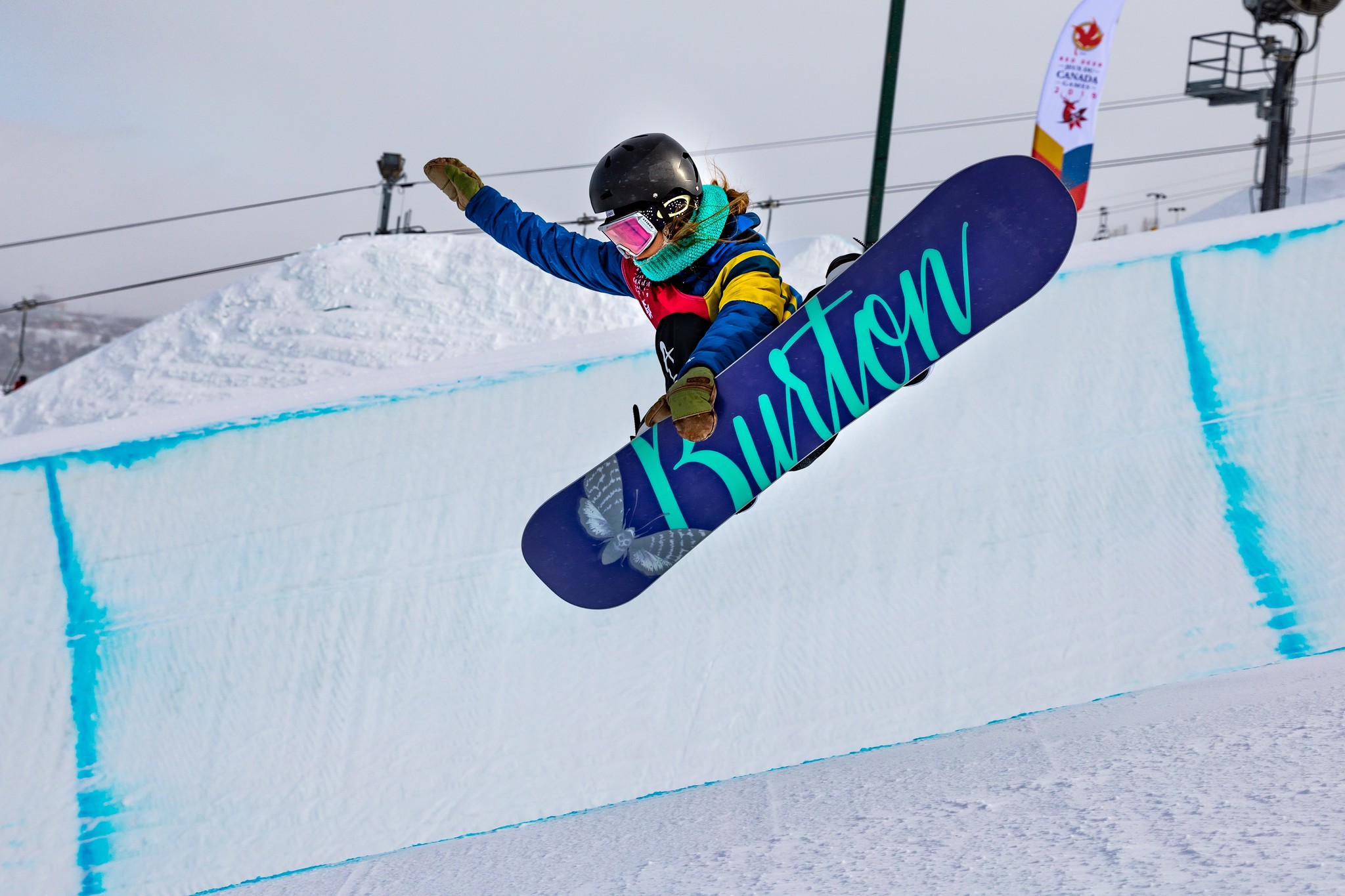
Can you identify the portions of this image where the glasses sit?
[597,211,658,260]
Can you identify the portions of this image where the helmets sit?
[589,134,703,213]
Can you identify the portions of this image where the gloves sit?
[644,366,718,441]
[423,158,485,211]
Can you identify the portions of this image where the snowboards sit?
[519,155,1078,610]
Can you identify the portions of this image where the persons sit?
[424,135,933,510]
